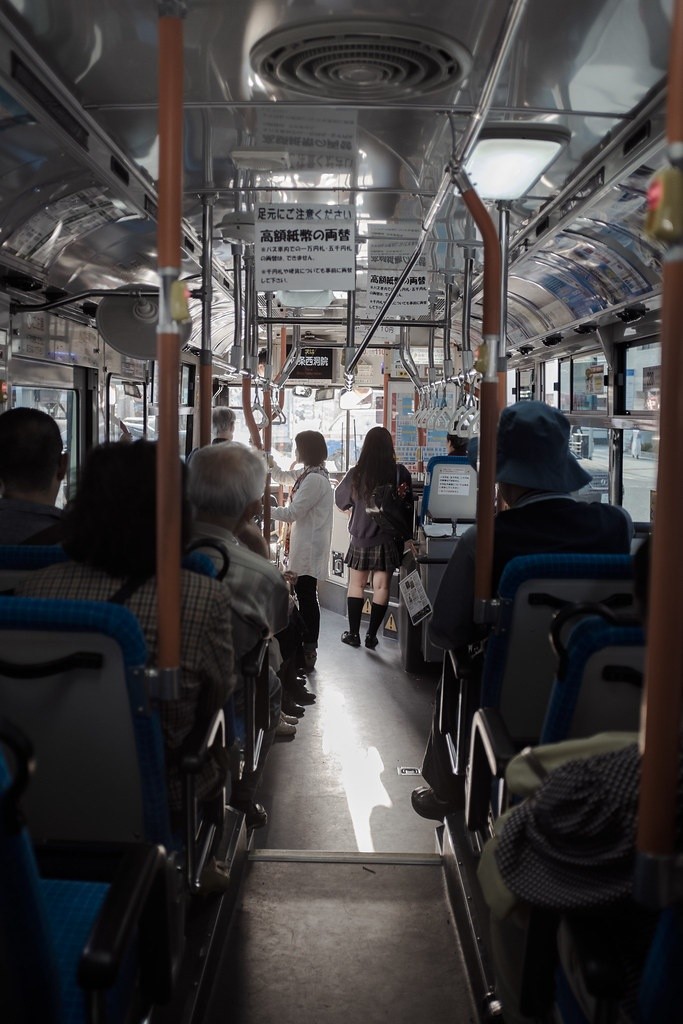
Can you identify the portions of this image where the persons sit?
[11,435,238,898]
[444,419,469,457]
[257,429,332,674]
[333,426,414,648]
[0,406,70,544]
[179,441,317,833]
[210,405,236,446]
[409,400,636,833]
[493,537,652,1023]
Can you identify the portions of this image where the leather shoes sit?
[365,633,379,647]
[279,701,305,716]
[411,787,468,822]
[340,631,361,647]
[290,681,316,701]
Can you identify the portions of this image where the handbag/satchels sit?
[366,483,417,539]
[477,730,647,927]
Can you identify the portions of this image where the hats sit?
[468,402,593,493]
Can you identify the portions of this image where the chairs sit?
[0,454,682,1024]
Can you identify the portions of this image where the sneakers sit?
[276,716,296,736]
[279,709,299,725]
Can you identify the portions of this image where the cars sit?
[568,448,624,505]
[40,395,378,481]
